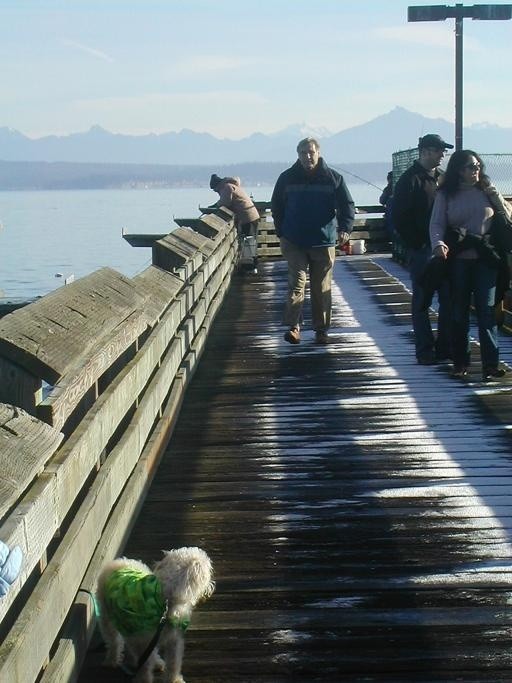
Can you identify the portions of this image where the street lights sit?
[407,3,512,152]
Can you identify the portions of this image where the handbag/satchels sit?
[493,210,512,252]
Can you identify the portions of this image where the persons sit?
[391,133,454,365]
[269,136,355,345]
[379,170,396,241]
[427,149,511,380]
[209,172,260,274]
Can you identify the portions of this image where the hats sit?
[210,174,220,189]
[419,134,454,148]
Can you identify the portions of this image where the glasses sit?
[464,162,480,170]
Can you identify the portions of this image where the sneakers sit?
[285,329,300,344]
[316,331,328,343]
[483,366,505,378]
[419,352,433,365]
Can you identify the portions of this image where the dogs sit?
[96,547,217,683]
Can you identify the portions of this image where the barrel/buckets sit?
[350,240,367,254]
